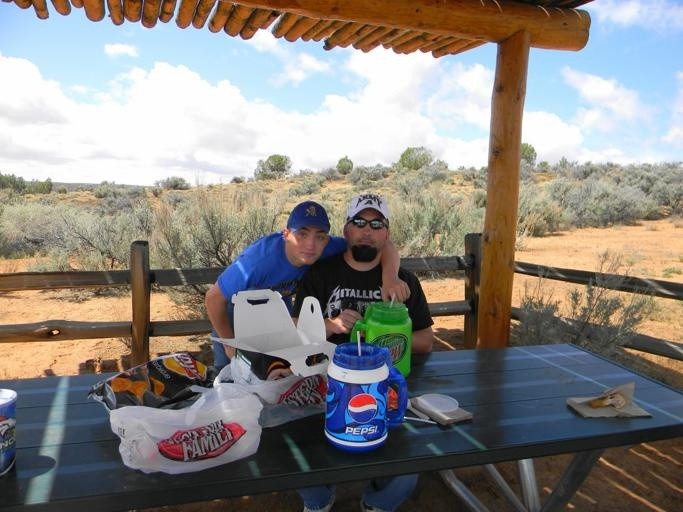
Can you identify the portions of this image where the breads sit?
[591,392,626,409]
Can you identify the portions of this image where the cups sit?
[324,343,408,452]
[351,300,412,379]
[0,387,18,476]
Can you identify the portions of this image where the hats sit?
[286,201,330,235]
[344,194,387,220]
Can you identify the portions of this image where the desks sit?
[0,344,683,512]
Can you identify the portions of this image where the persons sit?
[205,199,412,374]
[292,192,435,512]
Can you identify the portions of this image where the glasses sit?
[352,217,387,232]
